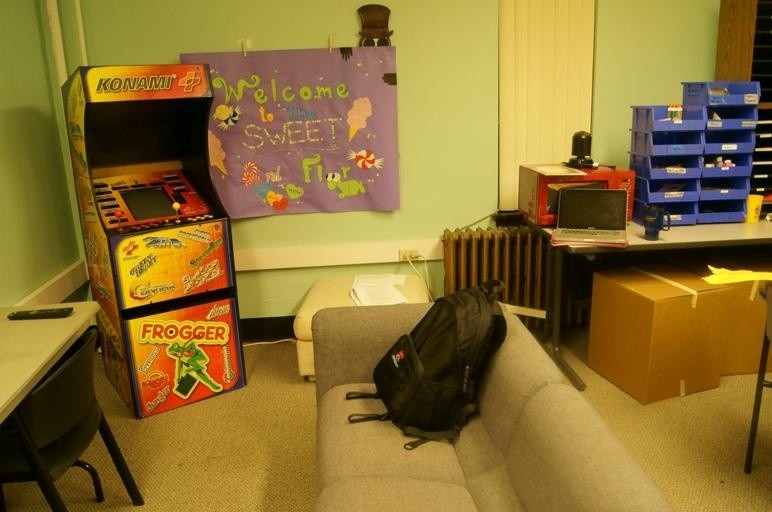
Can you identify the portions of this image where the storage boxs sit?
[690,262,772,376]
[587,269,733,406]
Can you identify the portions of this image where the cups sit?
[747,194,764,227]
[643,206,671,241]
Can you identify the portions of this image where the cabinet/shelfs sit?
[628,82,761,227]
[717,15,772,220]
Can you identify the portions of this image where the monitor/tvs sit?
[116,182,183,221]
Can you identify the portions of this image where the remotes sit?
[7,307,74,320]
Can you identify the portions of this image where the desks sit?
[1,299,106,504]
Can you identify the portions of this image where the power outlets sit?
[399,249,420,262]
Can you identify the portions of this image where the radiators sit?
[440,226,547,338]
[499,0,595,214]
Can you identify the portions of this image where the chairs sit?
[743,282,772,475]
[0,327,145,512]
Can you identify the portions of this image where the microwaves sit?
[518,163,635,226]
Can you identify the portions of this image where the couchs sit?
[311,303,669,510]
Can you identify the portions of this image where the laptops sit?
[550,186,630,249]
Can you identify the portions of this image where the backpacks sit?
[346,280,507,451]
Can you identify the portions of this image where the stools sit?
[291,276,431,381]
